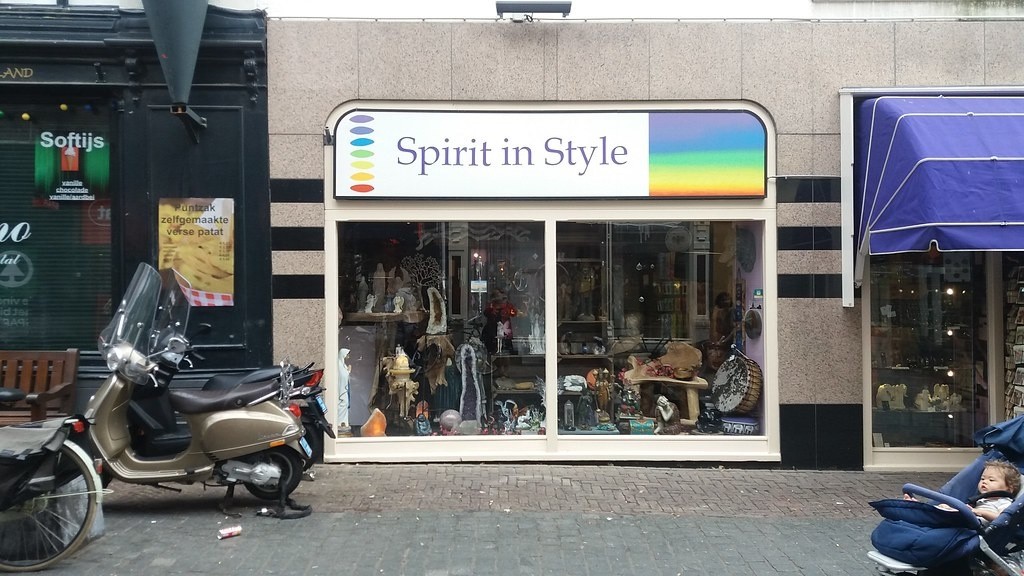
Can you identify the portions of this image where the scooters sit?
[124,268,336,482]
[69,262,316,517]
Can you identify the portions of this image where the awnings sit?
[854,96,1024,255]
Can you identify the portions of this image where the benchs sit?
[0,348,81,428]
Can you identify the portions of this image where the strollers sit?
[866,416,1024,576]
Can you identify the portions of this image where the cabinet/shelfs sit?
[490,237,711,426]
[870,252,975,448]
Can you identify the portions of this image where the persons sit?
[592,368,623,421]
[337,349,352,427]
[654,396,681,435]
[578,261,595,316]
[393,293,401,311]
[711,291,733,342]
[415,413,432,436]
[359,276,368,310]
[365,295,376,311]
[495,322,504,353]
[481,292,518,351]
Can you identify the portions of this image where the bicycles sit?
[0,382,103,572]
